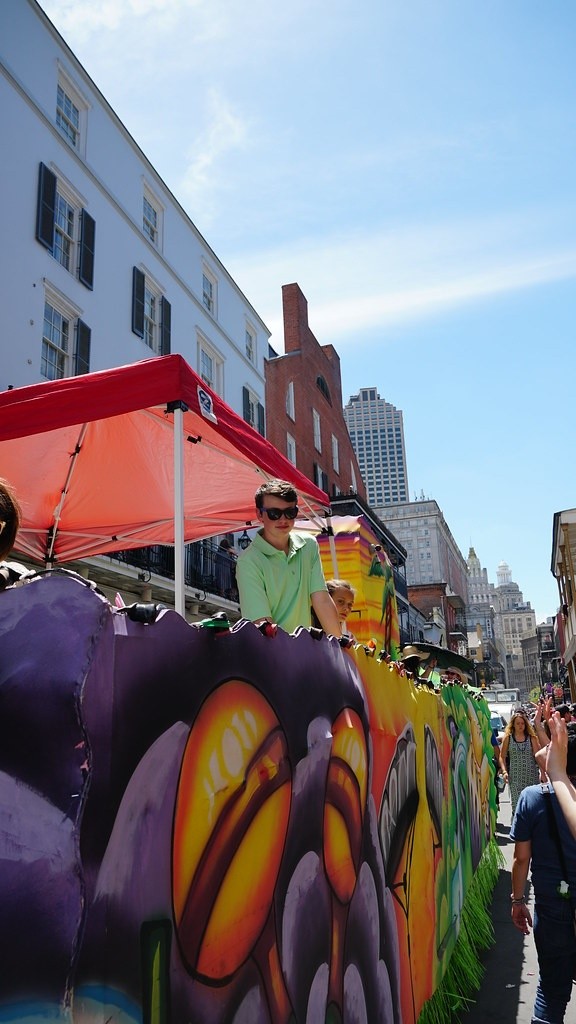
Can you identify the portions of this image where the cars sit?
[491,711,508,750]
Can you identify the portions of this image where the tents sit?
[1,353,341,621]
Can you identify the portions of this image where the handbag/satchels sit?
[495,771,506,792]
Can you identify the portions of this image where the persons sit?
[445,667,468,686]
[236,480,342,638]
[492,695,576,1024]
[0,484,28,591]
[315,580,371,655]
[396,646,438,682]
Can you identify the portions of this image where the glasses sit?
[261,505,299,520]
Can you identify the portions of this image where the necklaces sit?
[515,740,527,751]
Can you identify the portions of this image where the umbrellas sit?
[404,642,475,681]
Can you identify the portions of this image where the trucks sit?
[480,689,523,719]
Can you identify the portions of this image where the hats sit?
[554,704,574,717]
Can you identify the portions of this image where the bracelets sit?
[429,665,433,669]
[511,894,526,904]
[502,771,508,775]
